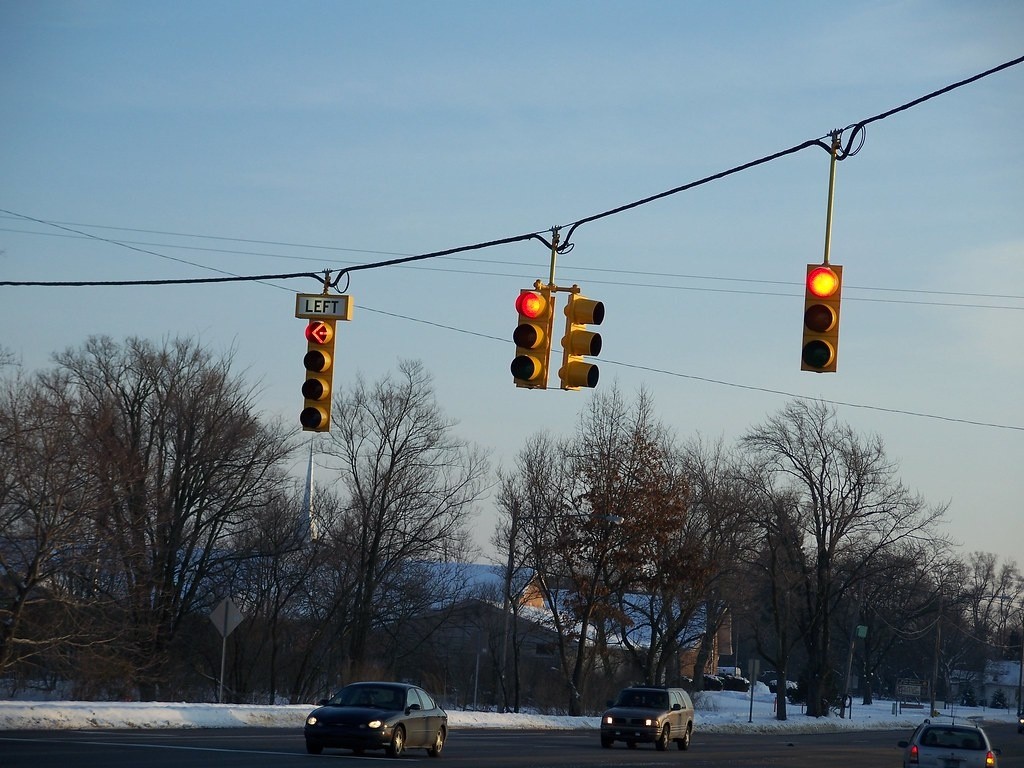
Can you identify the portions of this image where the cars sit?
[304,681,448,759]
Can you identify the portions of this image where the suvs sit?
[600,684,694,751]
[898,718,1002,768]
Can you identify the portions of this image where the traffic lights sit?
[300,318,336,432]
[510,287,556,390]
[801,264,843,374]
[558,292,606,392]
